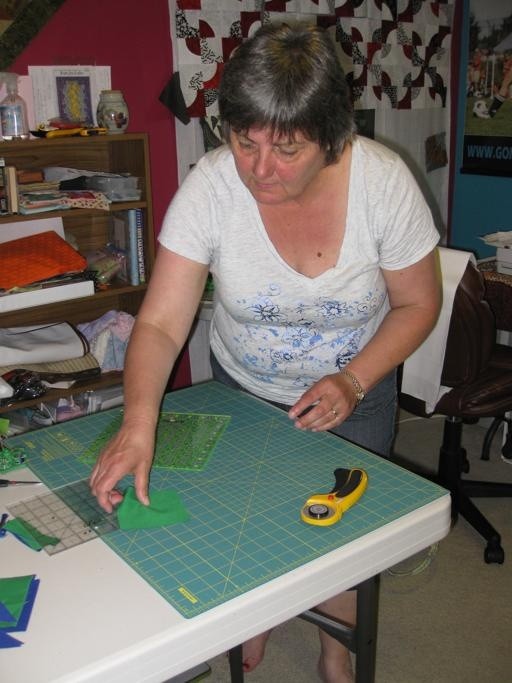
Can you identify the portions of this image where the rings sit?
[330,407,339,418]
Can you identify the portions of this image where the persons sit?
[88,24,447,682]
[466,44,511,120]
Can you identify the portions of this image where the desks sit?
[474,255,511,365]
[0,382,453,682]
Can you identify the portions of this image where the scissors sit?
[0,479,45,487]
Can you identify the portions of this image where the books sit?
[135,208,146,283]
[111,209,139,287]
[8,166,17,213]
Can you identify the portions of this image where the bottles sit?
[0,73,30,141]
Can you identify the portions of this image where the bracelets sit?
[342,367,366,405]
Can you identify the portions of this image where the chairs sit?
[396,247,512,564]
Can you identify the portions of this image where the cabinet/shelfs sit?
[0,133,159,413]
